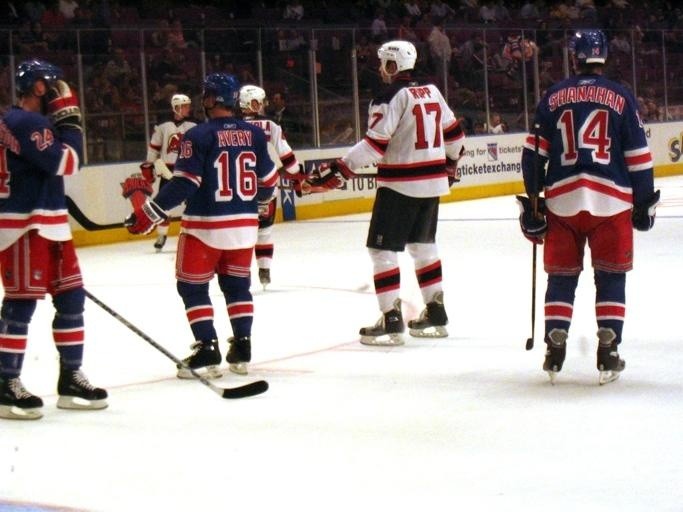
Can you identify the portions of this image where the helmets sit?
[170,94,192,106]
[376,40,418,72]
[14,58,64,97]
[567,27,609,64]
[238,84,266,109]
[199,71,240,107]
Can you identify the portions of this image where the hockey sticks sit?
[85,290,269,399]
[266,142,460,183]
[154,158,295,189]
[526,124,539,350]
[65,195,181,231]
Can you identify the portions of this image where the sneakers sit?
[225,336,251,363]
[258,268,271,285]
[176,338,222,369]
[153,235,168,249]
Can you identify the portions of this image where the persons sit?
[145,93,197,249]
[305,40,467,336]
[1,1,682,166]
[0,60,107,408]
[517,28,659,370]
[237,85,304,282]
[125,73,279,369]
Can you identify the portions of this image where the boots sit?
[408,290,449,329]
[57,357,107,400]
[359,298,405,337]
[542,328,568,372]
[0,372,43,409]
[596,326,626,372]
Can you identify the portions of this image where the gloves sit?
[632,189,661,233]
[515,195,548,245]
[124,196,169,236]
[43,78,82,126]
[284,158,357,197]
[140,161,156,183]
[444,155,458,188]
